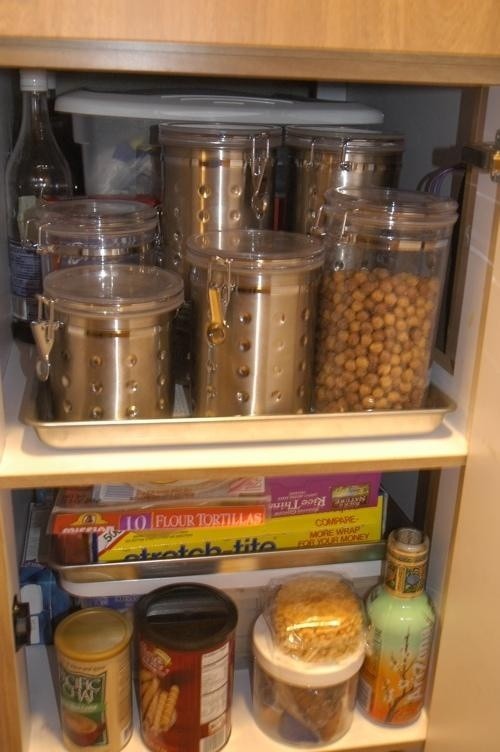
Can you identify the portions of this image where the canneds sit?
[52,607,132,751]
[137,583,237,752]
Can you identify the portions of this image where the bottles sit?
[179,227,325,419]
[5,70,77,343]
[249,613,366,750]
[282,117,406,277]
[22,192,161,282]
[154,122,281,363]
[356,527,438,728]
[29,266,189,420]
[309,184,457,412]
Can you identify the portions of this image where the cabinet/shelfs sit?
[0,0,499,751]
